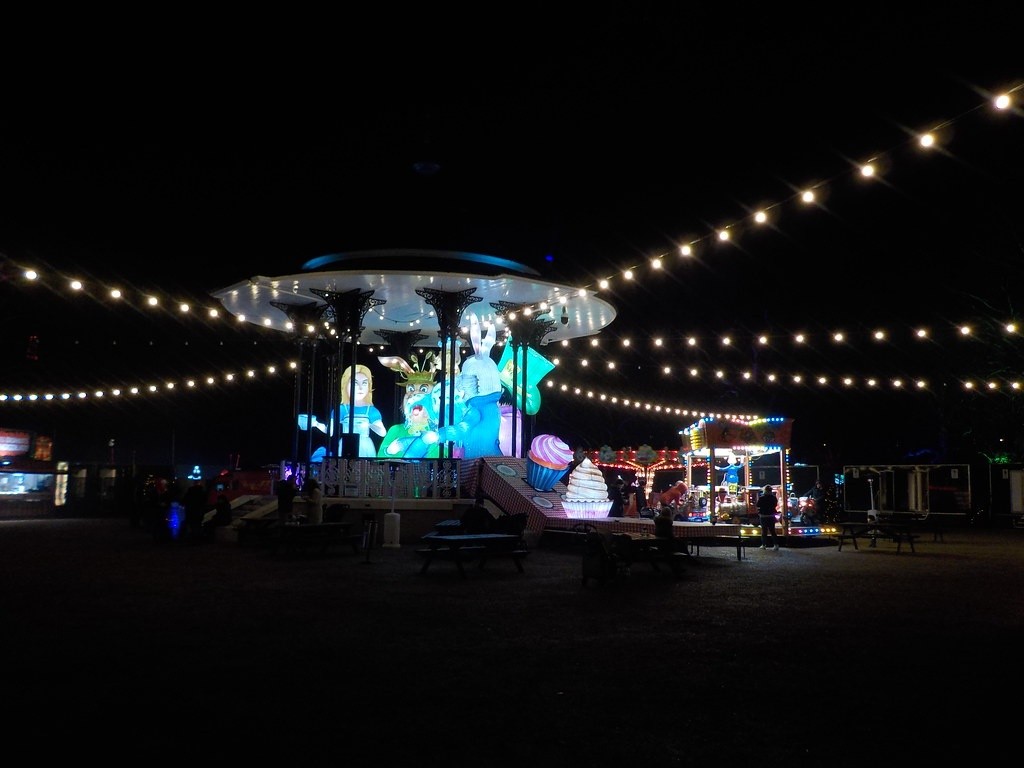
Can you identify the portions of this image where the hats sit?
[719,488,727,493]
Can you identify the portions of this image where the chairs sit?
[572,523,618,579]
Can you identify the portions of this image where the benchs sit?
[270,533,363,559]
[415,548,529,576]
[837,522,920,552]
[834,534,920,552]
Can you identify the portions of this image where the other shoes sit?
[760,545,766,549]
[773,545,779,550]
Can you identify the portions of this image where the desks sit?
[610,532,667,572]
[424,535,523,576]
[274,522,356,559]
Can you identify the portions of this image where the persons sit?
[136,474,325,544]
[606,474,827,563]
